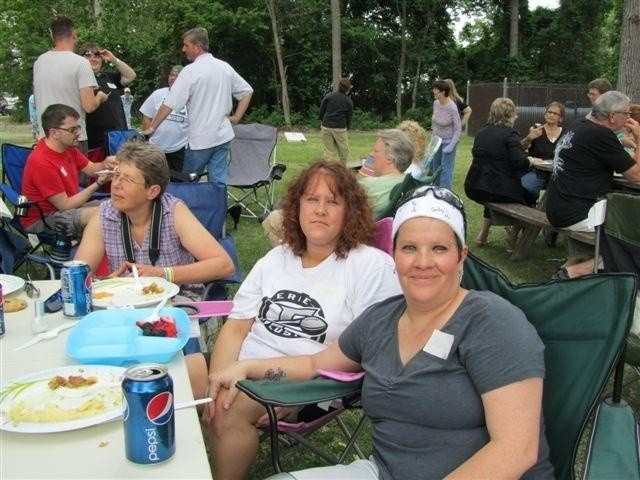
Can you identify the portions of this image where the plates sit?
[0,364,127,433]
[0,274,25,295]
[92,277,180,307]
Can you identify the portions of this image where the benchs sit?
[483,200,597,244]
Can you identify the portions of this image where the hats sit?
[391,191,466,248]
[171,65,184,72]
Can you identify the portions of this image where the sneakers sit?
[544,227,558,247]
[475,237,514,248]
[550,267,570,281]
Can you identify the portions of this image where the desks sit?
[508,159,640,260]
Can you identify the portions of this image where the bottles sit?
[47,236,71,279]
[31,300,48,332]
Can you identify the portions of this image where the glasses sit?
[395,186,468,242]
[44,288,63,313]
[23,280,40,299]
[84,51,104,57]
[54,125,81,132]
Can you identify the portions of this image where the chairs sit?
[223,123,286,225]
[591,193,640,407]
[0,143,110,280]
[24,183,243,371]
[104,129,199,183]
[173,217,395,466]
[235,252,640,480]
[371,134,442,223]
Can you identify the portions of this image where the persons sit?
[262,79,472,249]
[28,17,190,183]
[464,97,546,252]
[185,161,404,480]
[521,78,640,280]
[18,104,119,242]
[201,184,557,479]
[71,140,236,285]
[141,26,254,185]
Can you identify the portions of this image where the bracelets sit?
[163,266,174,283]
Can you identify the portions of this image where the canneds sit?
[121,363,178,468]
[60,259,93,319]
[0,283,5,338]
